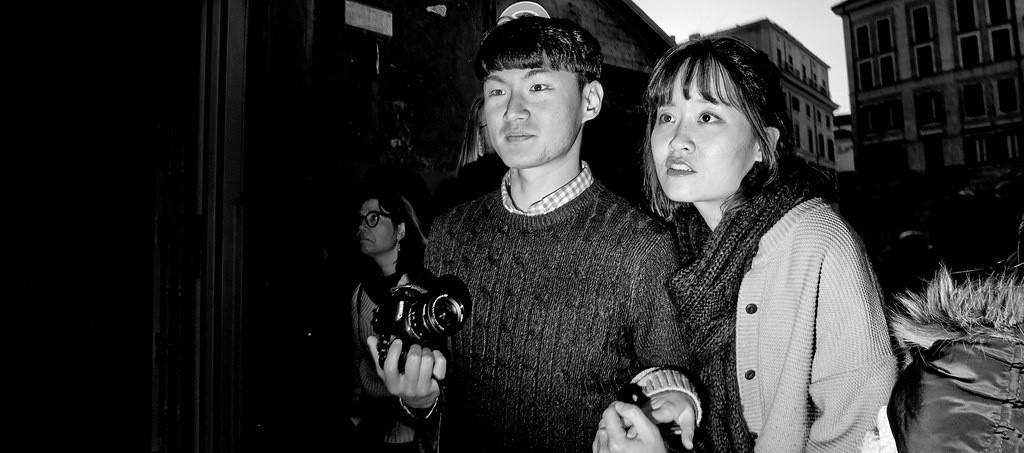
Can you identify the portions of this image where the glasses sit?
[352,211,395,229]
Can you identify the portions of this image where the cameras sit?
[372,285,467,373]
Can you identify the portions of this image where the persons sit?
[593,36,898,453]
[350,14,1024,453]
[351,13,702,453]
